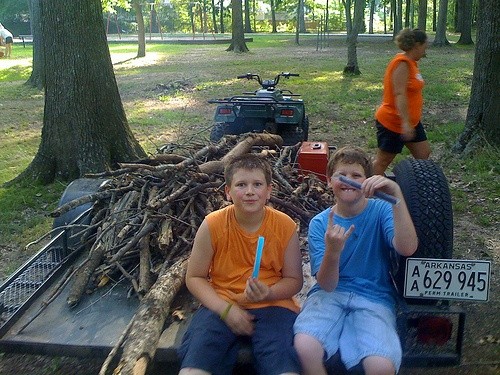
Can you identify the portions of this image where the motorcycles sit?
[206,72,339,193]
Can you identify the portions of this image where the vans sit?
[0,22,13,42]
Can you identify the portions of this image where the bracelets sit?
[221,304,232,321]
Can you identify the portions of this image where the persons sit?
[292,146,418,375]
[178,154,304,375]
[0,29,13,57]
[375,28,430,177]
